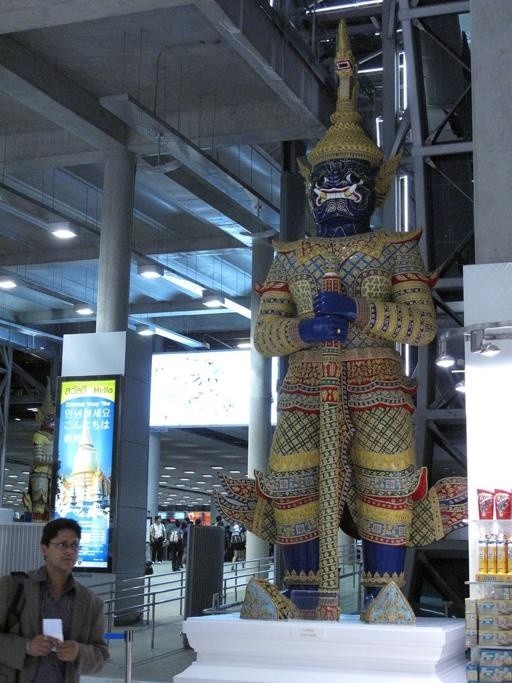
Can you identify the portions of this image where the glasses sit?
[50,543,80,551]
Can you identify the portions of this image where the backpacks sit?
[230,532,242,544]
[170,529,180,544]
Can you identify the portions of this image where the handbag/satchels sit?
[150,524,155,543]
[162,538,169,547]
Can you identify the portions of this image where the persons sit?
[249,121,441,621]
[1,517,111,683]
[150,514,244,571]
[29,404,56,522]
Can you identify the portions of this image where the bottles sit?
[478,532,512,575]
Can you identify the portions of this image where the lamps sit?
[0,220,225,337]
[435,320,512,392]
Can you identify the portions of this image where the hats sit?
[233,525,239,532]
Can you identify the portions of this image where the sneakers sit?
[231,563,245,570]
[173,564,183,571]
[152,561,162,564]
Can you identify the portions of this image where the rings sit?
[43,634,49,640]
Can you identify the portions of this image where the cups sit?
[23,511,33,522]
[213,593,220,610]
